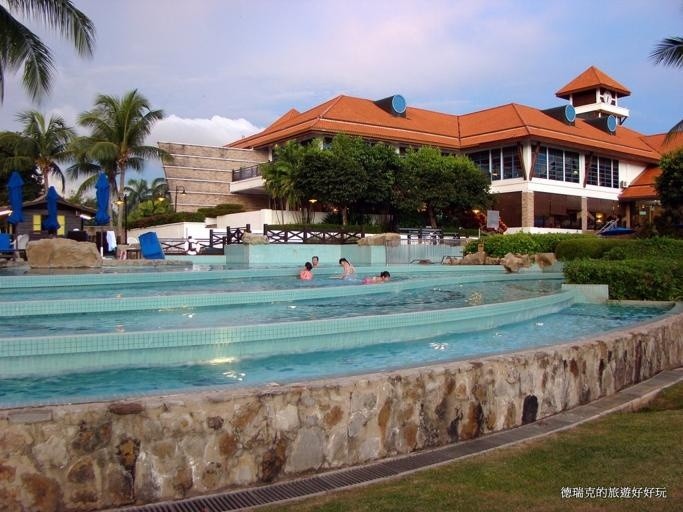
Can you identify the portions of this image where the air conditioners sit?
[621,181,628,188]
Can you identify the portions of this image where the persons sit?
[300,262,313,280]
[358,271,390,284]
[312,256,319,267]
[339,258,356,280]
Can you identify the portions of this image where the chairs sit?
[0,232,29,257]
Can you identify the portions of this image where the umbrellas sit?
[7,171,25,250]
[43,186,60,237]
[95,170,111,258]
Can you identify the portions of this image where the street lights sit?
[114,196,129,245]
[175,185,188,213]
